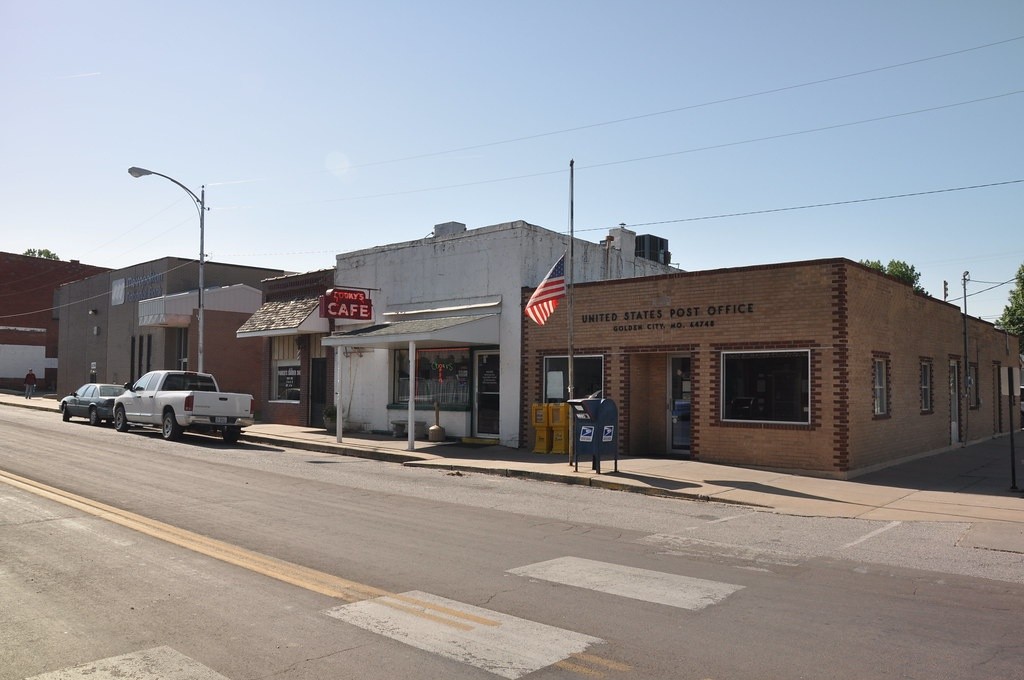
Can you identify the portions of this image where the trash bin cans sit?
[567,398,618,474]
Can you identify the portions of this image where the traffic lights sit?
[944,281,948,298]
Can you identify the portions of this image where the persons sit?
[24,368,37,399]
[525,250,568,327]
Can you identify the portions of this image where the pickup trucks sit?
[112,370,255,445]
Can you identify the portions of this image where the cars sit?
[58,383,128,424]
[399,377,434,403]
[277,387,301,403]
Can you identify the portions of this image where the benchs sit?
[390,420,426,440]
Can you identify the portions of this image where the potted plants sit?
[322,404,336,436]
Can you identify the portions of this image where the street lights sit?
[127,166,206,373]
[962,270,970,385]
